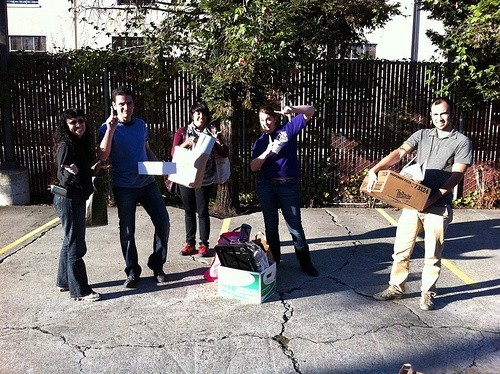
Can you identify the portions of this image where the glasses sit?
[66,118,85,125]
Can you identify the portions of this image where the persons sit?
[251,105,321,277]
[48,108,108,302]
[171,103,230,256]
[96,87,171,288]
[366,97,473,310]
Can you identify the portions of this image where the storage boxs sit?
[217,262,278,305]
[357,170,432,214]
[138,159,178,176]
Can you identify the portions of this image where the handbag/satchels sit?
[211,127,230,183]
[168,143,206,188]
[84,175,108,228]
[203,226,248,282]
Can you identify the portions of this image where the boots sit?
[295,244,319,277]
[269,240,281,266]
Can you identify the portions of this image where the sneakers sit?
[198,245,209,257]
[180,244,196,256]
[72,291,102,302]
[124,276,140,288]
[373,287,405,301]
[154,270,167,282]
[420,291,436,311]
[58,286,70,292]
